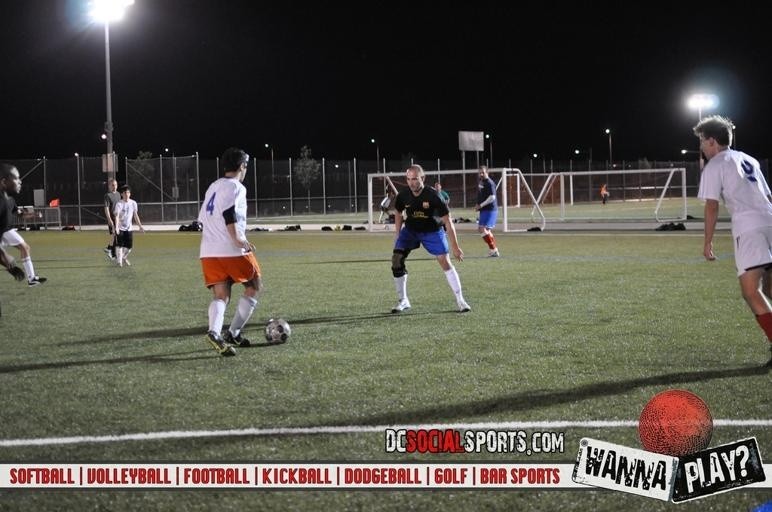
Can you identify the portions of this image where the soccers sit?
[264,317,289,343]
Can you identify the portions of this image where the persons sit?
[692,115,772,368]
[198,147,263,357]
[391,164,471,314]
[379,197,392,224]
[104,178,121,261]
[0,163,25,281]
[111,184,144,268]
[435,183,450,231]
[473,165,500,257]
[601,184,609,205]
[2,196,48,288]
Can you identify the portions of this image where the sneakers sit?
[391,296,412,313]
[104,247,131,267]
[206,330,237,357]
[457,298,471,313]
[483,248,500,259]
[222,330,251,348]
[27,276,48,287]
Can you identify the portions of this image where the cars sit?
[14,205,46,224]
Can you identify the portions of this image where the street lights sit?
[604,128,613,165]
[74,152,85,183]
[686,93,719,169]
[85,0,137,179]
[165,146,175,179]
[263,143,279,215]
[370,137,381,172]
[486,133,495,167]
